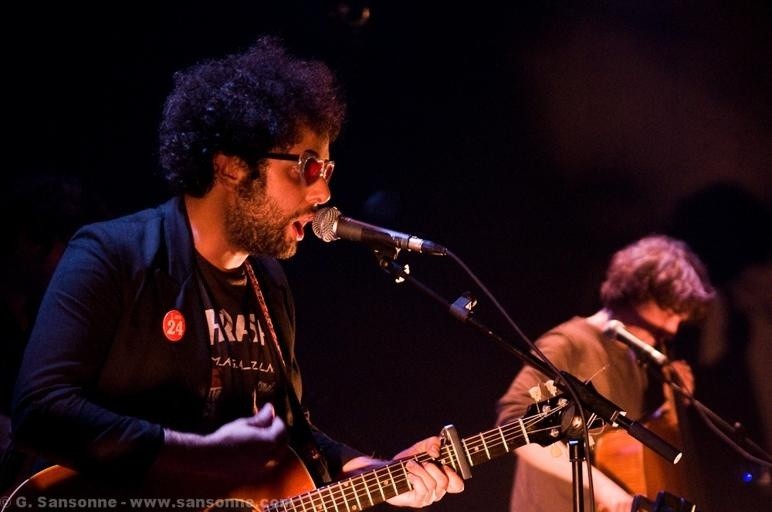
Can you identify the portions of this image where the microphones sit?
[602,319,668,367]
[313,207,443,261]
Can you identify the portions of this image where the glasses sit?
[266,149,335,188]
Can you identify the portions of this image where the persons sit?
[9,44,466,511]
[496,234,717,510]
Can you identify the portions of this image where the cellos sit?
[594,332,708,512]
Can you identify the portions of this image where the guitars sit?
[0,381,605,512]
